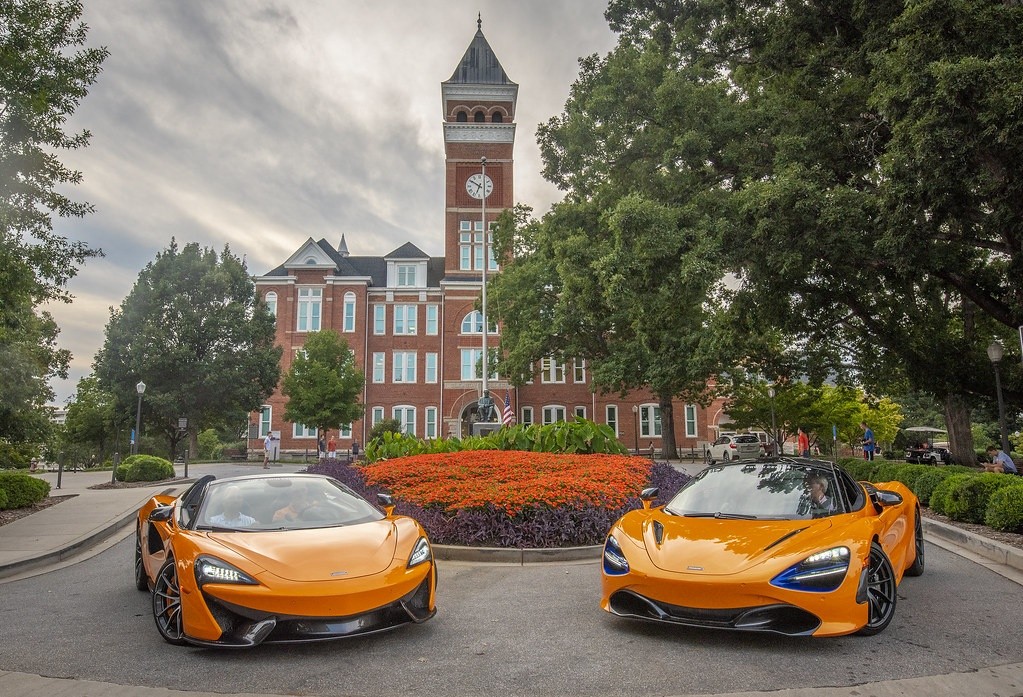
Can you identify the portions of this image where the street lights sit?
[986,339,1011,457]
[767,387,777,457]
[632,404,639,453]
[133,380,147,454]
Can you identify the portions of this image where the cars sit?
[904,440,951,464]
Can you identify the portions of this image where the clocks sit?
[466,174,494,198]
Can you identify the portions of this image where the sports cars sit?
[599,458,925,637]
[134,473,439,651]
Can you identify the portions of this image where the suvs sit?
[706,432,765,465]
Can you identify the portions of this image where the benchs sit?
[632,450,652,458]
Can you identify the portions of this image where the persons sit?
[860,422,874,460]
[263,431,271,469]
[798,427,809,458]
[319,434,327,461]
[327,436,337,457]
[649,441,655,458]
[205,486,259,526]
[981,446,1017,475]
[351,439,359,460]
[478,389,494,422]
[802,475,834,509]
[273,482,327,523]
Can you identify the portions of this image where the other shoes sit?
[263,466,269,469]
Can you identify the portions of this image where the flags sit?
[503,389,514,426]
